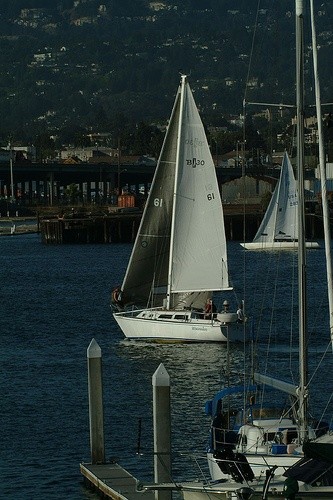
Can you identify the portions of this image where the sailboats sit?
[111,71,255,343]
[144,0,333,500]
[239,150,320,251]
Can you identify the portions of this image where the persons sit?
[110,286,125,312]
[203,299,216,319]
[236,305,244,323]
[258,408,271,419]
